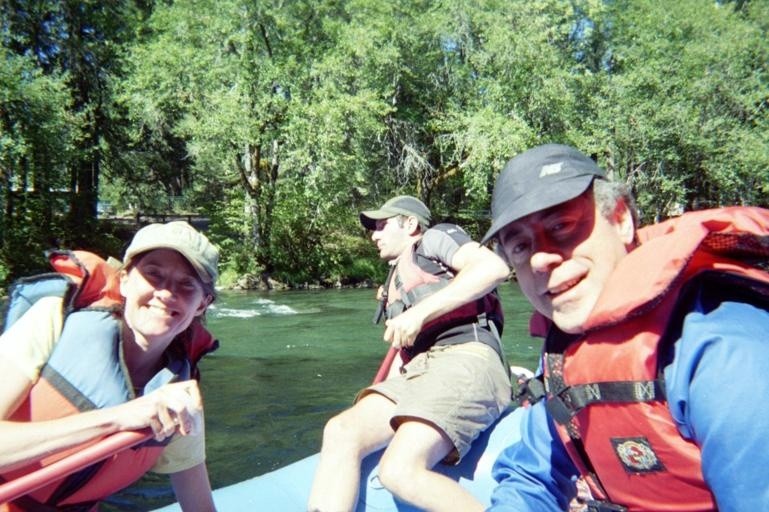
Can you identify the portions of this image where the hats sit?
[359,193,434,230]
[479,143,612,245]
[120,220,221,284]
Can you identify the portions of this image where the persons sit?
[304,193,516,512]
[478,144,769,511]
[1,220,221,511]
